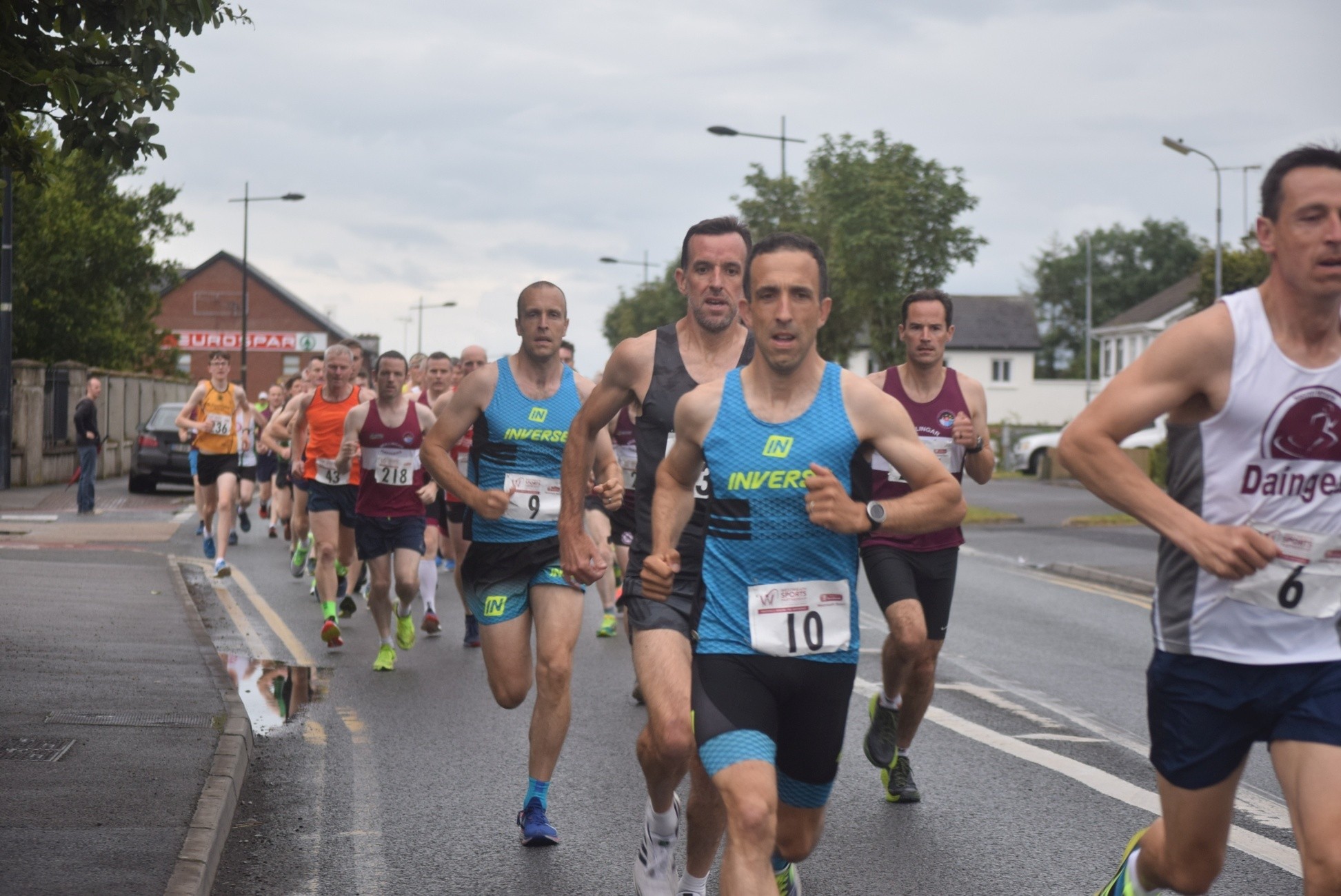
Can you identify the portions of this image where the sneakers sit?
[420,612,442,634]
[338,596,356,618]
[863,692,903,768]
[464,614,480,647]
[596,612,617,638]
[1094,827,1186,896]
[616,594,624,607]
[334,559,349,597]
[392,599,416,650]
[880,747,921,803]
[290,532,315,578]
[516,796,559,846]
[320,615,342,648]
[373,644,397,672]
[675,881,706,896]
[771,854,795,896]
[633,790,682,896]
[628,679,647,706]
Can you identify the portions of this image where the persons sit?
[558,216,754,895]
[556,339,641,706]
[850,290,995,802]
[175,337,491,670]
[640,233,968,896]
[74,379,107,516]
[1058,146,1340,896]
[419,281,625,845]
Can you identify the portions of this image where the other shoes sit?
[258,498,268,519]
[443,558,456,572]
[281,518,291,540]
[236,504,251,532]
[228,532,238,545]
[76,508,103,517]
[196,519,204,535]
[214,560,228,579]
[203,536,216,558]
[268,525,277,538]
[306,548,316,577]
[435,556,444,568]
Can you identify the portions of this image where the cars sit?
[127,402,193,494]
[1008,411,1172,480]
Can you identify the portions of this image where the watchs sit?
[863,500,887,532]
[965,435,984,455]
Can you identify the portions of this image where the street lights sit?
[704,115,805,183]
[598,252,660,293]
[409,299,461,355]
[230,187,304,391]
[1160,137,1224,305]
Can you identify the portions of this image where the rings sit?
[609,498,612,504]
